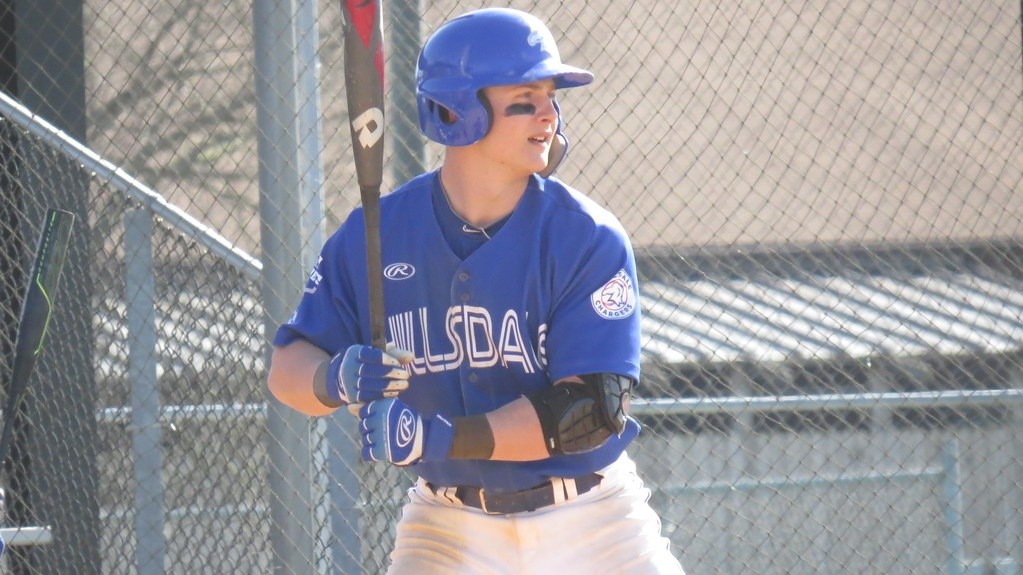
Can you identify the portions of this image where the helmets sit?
[415,7,594,179]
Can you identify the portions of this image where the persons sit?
[266,7,688,575]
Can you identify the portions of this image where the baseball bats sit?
[0,206,76,467]
[338,0,386,353]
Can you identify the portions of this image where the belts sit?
[428,473,603,514]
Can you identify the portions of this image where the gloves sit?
[347,397,455,467]
[326,342,415,408]
